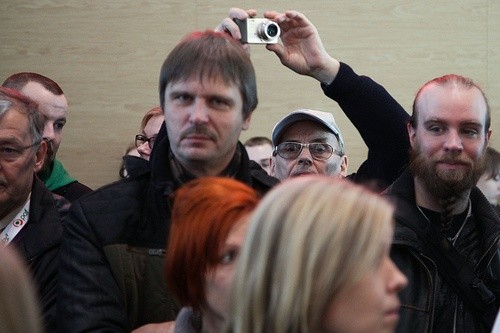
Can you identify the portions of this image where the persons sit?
[242,136,275,175]
[0,239,42,332]
[474,147,500,207]
[214,8,412,194]
[58,30,282,332]
[233,175,408,332]
[120,142,141,180]
[0,86,72,333]
[380,74,500,333]
[129,177,261,332]
[135,107,166,162]
[0,72,95,205]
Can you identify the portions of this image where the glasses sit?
[135,134,157,149]
[272,142,344,161]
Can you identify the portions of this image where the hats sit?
[271,109,344,147]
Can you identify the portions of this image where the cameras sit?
[234,17,281,45]
